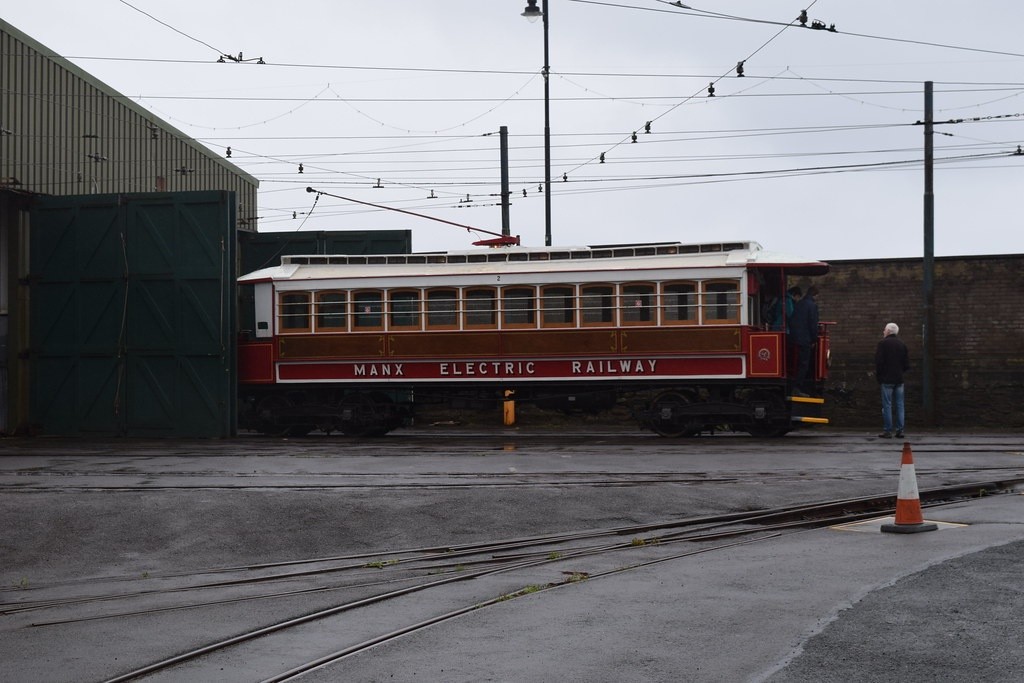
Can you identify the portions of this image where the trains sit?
[237,237,834,440]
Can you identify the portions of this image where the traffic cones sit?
[879,439,940,535]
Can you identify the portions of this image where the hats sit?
[808,287,819,296]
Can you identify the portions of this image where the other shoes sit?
[879,432,892,438]
[895,432,904,438]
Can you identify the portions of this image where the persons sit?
[765,284,821,397]
[875,322,909,438]
